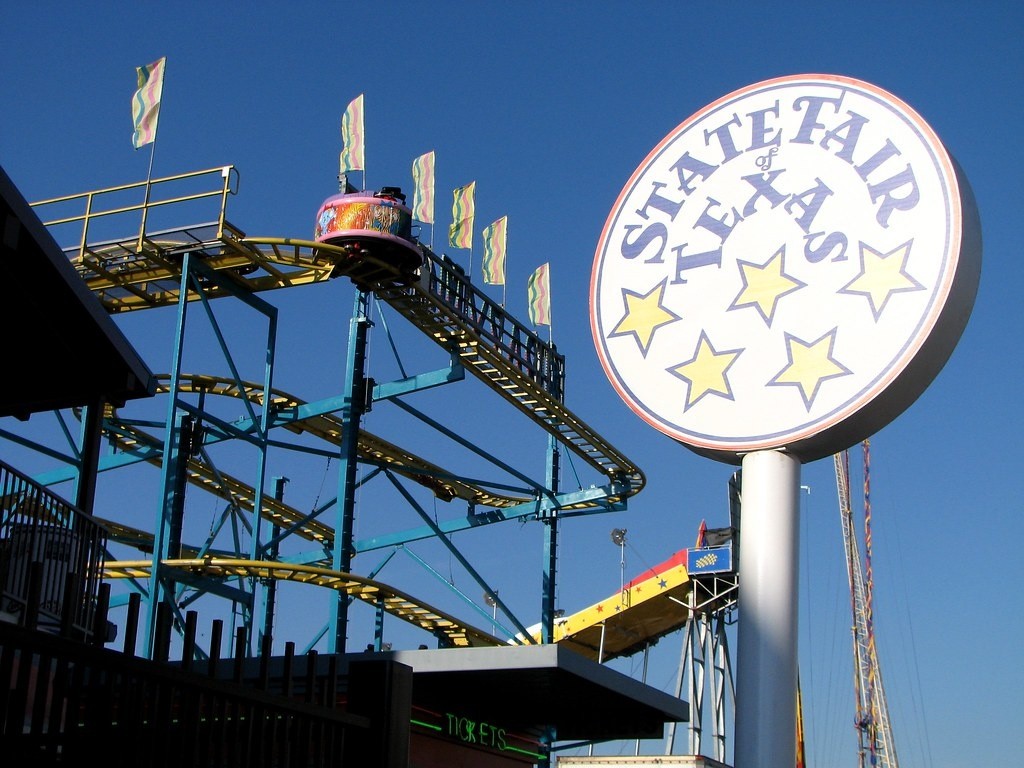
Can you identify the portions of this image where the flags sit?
[340,93,365,175]
[448,180,476,249]
[131,57,166,151]
[482,215,508,286]
[412,149,435,225]
[527,262,551,327]
